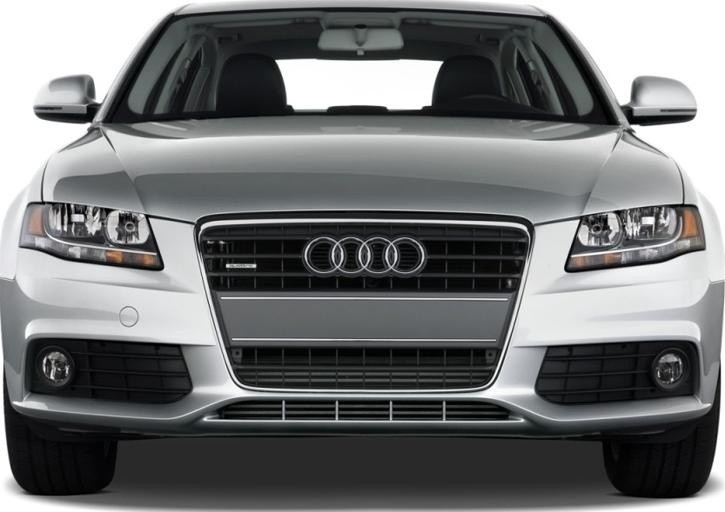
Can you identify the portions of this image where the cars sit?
[0,0,724,496]
